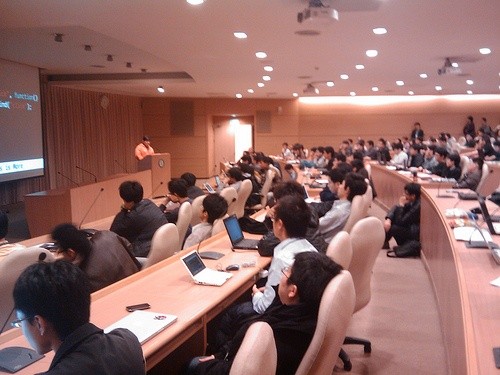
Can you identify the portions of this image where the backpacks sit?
[386,240,421,258]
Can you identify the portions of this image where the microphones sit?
[0,252,46,373]
[58,171,83,186]
[148,181,163,198]
[78,187,104,228]
[76,166,97,182]
[115,159,129,174]
[197,197,237,260]
[446,168,493,217]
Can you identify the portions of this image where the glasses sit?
[11,318,33,328]
[271,217,278,221]
[281,266,293,284]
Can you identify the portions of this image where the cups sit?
[403,159,408,170]
[267,192,274,208]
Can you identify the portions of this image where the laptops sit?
[223,214,259,249]
[181,250,232,287]
[203,182,216,194]
[472,223,500,264]
[214,175,226,189]
[103,310,178,344]
[478,196,500,235]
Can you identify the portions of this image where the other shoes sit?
[382,242,390,249]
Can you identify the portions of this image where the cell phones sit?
[126,303,150,312]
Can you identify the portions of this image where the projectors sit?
[296,6,339,25]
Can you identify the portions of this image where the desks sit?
[419,185,500,375]
[0,204,274,375]
[24,170,152,238]
[370,161,456,214]
[270,156,330,198]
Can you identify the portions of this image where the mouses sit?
[226,264,239,271]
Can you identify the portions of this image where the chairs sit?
[0,137,472,375]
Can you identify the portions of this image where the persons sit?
[186,178,344,375]
[11,257,146,375]
[0,202,144,336]
[109,172,206,259]
[277,141,377,218]
[338,114,500,191]
[227,148,282,215]
[135,135,154,162]
[381,183,421,250]
